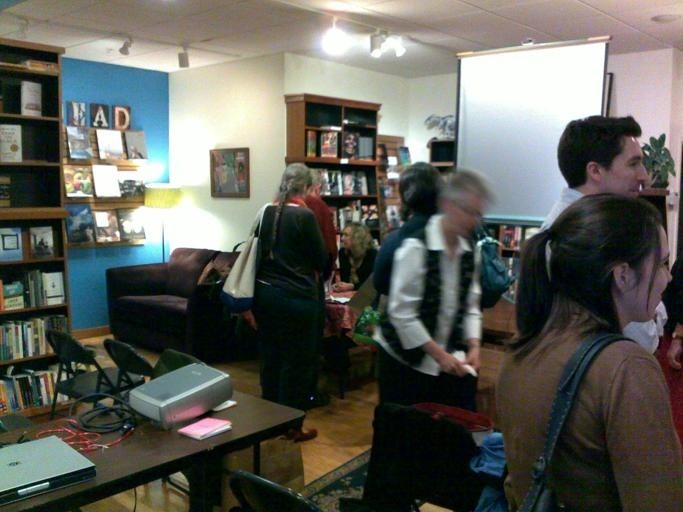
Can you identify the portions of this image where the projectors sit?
[128,361,233,431]
[0,434,97,507]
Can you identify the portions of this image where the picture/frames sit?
[208,146,250,199]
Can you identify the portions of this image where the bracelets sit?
[671,331,683,341]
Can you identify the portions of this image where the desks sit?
[0,370,306,512]
[323,291,376,397]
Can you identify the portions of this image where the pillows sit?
[197,258,220,285]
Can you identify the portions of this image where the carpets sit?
[297,446,425,511]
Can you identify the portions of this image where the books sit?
[482,221,540,307]
[0,59,68,418]
[174,416,234,442]
[64,125,147,244]
[304,112,410,271]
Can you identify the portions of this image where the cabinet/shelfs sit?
[380,135,411,239]
[284,92,383,247]
[0,39,74,425]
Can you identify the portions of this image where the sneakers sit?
[310,391,330,407]
[295,429,317,441]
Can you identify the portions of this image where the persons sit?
[247,162,494,443]
[492,191,682,512]
[662,246,683,374]
[536,113,671,358]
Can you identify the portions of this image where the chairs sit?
[104,337,154,408]
[229,465,325,512]
[44,329,145,421]
[147,348,200,382]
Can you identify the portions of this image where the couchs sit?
[106,248,241,364]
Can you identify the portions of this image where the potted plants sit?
[642,133,677,197]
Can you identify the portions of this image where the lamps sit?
[321,14,355,55]
[111,31,241,67]
[371,34,408,60]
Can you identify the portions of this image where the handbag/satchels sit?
[472,237,516,310]
[352,306,387,353]
[222,236,260,314]
[516,457,577,512]
